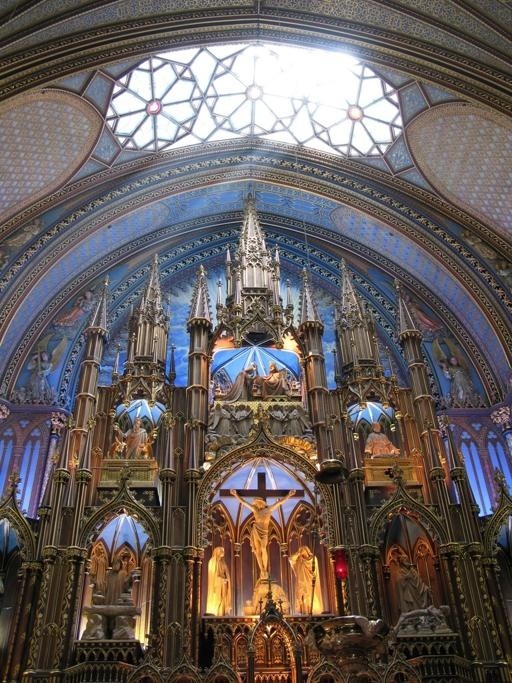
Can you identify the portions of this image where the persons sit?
[260,363,290,398]
[394,555,432,613]
[439,356,473,399]
[104,558,131,605]
[287,545,323,615]
[205,547,231,617]
[229,488,296,579]
[220,362,257,403]
[364,421,400,458]
[112,418,148,460]
[211,403,305,435]
